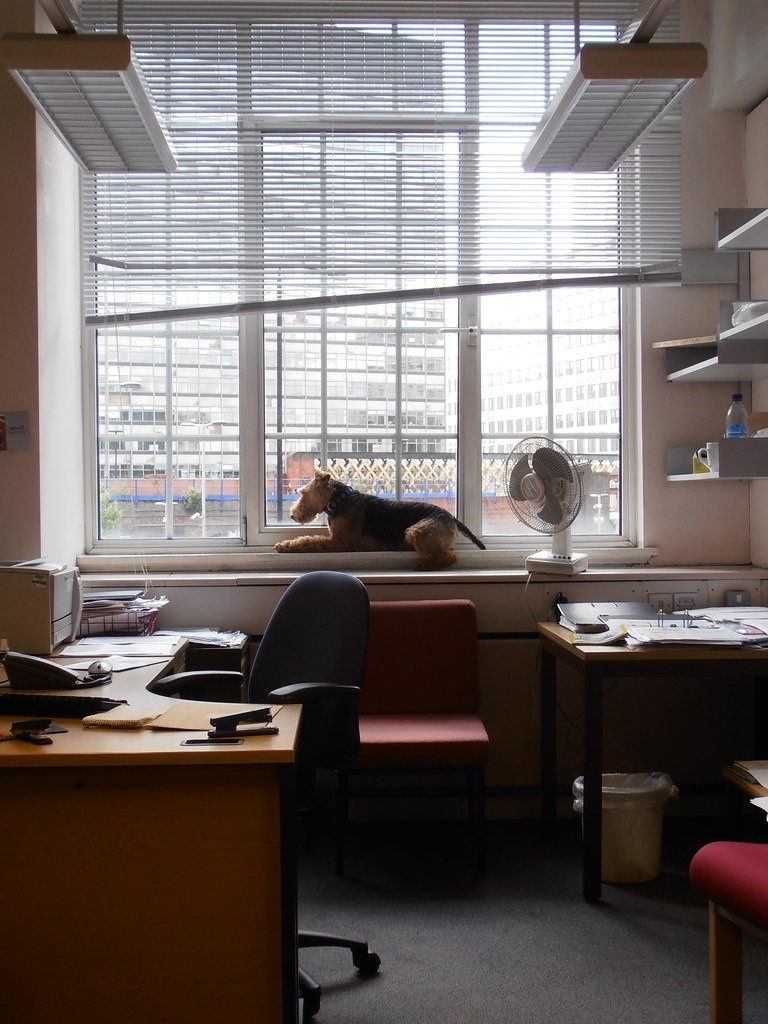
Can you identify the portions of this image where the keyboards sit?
[0,692,128,720]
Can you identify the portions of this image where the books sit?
[557,602,768,648]
[83,589,148,633]
[82,703,164,727]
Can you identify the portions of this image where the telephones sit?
[2,652,79,692]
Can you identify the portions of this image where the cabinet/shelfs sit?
[654,208,768,480]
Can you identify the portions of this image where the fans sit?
[505,432,591,577]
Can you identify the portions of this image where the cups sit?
[692,457,710,474]
[698,442,719,478]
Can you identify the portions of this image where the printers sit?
[0,559,83,655]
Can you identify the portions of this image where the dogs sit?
[272,467,486,571]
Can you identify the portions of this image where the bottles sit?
[725,394,748,439]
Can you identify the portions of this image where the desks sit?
[536,621,768,902]
[1,630,305,1024]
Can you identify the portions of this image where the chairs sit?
[152,571,381,1023]
[333,597,491,878]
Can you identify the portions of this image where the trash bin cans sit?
[571,770,678,886]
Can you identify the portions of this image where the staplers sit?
[207,706,279,736]
[9,718,52,745]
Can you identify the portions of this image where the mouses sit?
[88,661,113,675]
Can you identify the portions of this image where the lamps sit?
[0,1,178,175]
[524,1,705,174]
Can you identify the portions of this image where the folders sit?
[557,599,750,647]
